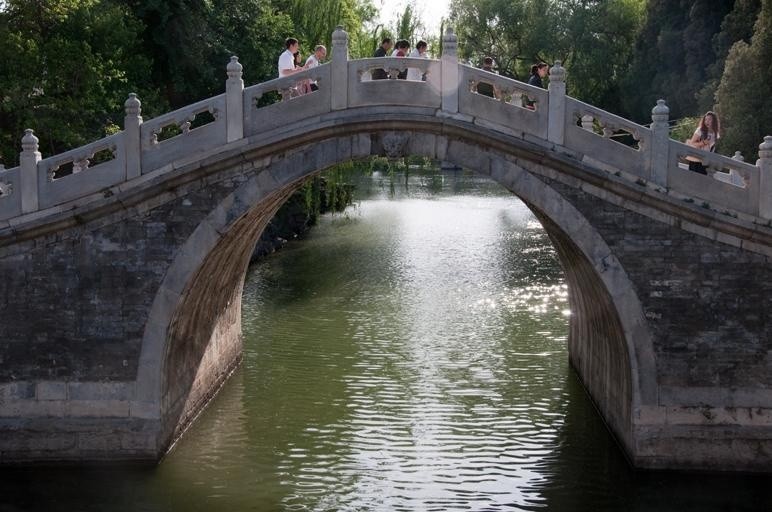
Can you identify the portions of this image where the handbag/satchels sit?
[685,139,701,162]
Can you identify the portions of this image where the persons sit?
[278,38,298,100]
[390,40,411,80]
[688,111,721,178]
[294,51,313,94]
[303,44,327,93]
[525,62,549,110]
[406,41,428,82]
[390,41,399,55]
[368,39,392,80]
[471,57,502,98]
